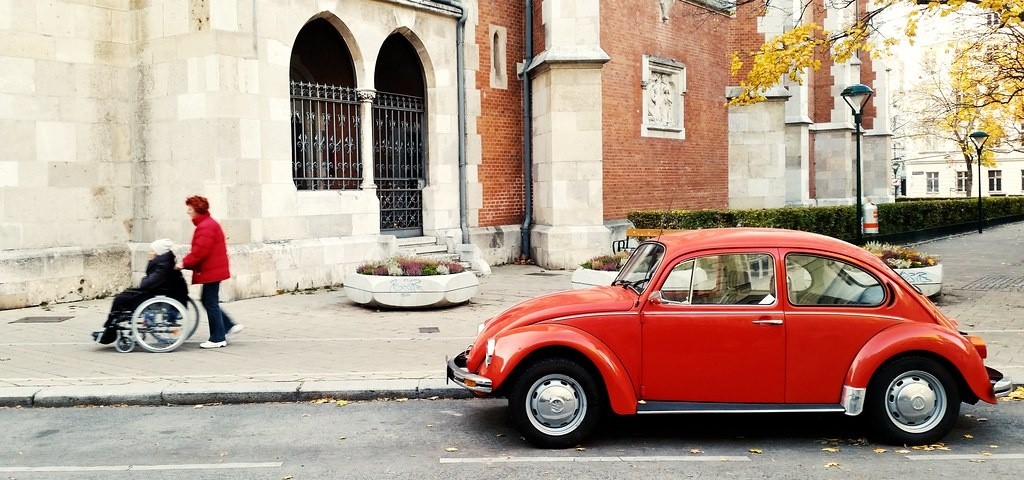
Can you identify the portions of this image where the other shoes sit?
[225,323,242,342]
[93,328,124,344]
[200,339,227,348]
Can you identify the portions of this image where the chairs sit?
[718,270,751,304]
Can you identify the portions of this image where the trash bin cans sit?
[862,203,879,234]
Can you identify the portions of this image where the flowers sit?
[357,251,466,277]
[579,252,631,272]
[861,240,942,268]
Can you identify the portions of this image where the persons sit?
[173,195,245,348]
[90,238,187,345]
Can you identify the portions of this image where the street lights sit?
[840,83,875,248]
[970,132,990,234]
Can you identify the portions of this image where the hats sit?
[150,238,174,255]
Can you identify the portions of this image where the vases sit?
[823,266,943,304]
[571,264,709,300]
[341,269,479,310]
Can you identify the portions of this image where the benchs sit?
[612,228,684,255]
[796,291,869,305]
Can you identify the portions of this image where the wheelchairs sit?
[91,265,200,353]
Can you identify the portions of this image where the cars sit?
[444,227,1013,451]
